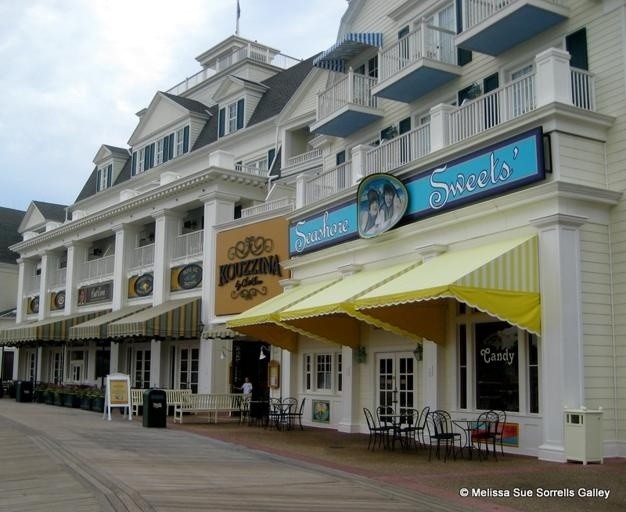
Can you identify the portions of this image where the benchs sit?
[174,394,244,424]
[122,389,193,420]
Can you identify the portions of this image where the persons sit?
[382,183,402,221]
[360,189,385,233]
[233,376,253,419]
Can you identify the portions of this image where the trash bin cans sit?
[142,389,166,428]
[16,381,33,403]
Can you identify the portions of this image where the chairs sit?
[363,405,506,462]
[237,395,305,432]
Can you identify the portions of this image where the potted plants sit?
[34,384,106,412]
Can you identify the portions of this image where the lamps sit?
[466,84,480,98]
[220,346,231,360]
[259,344,270,359]
[385,129,395,139]
[359,347,367,363]
[413,343,422,362]
